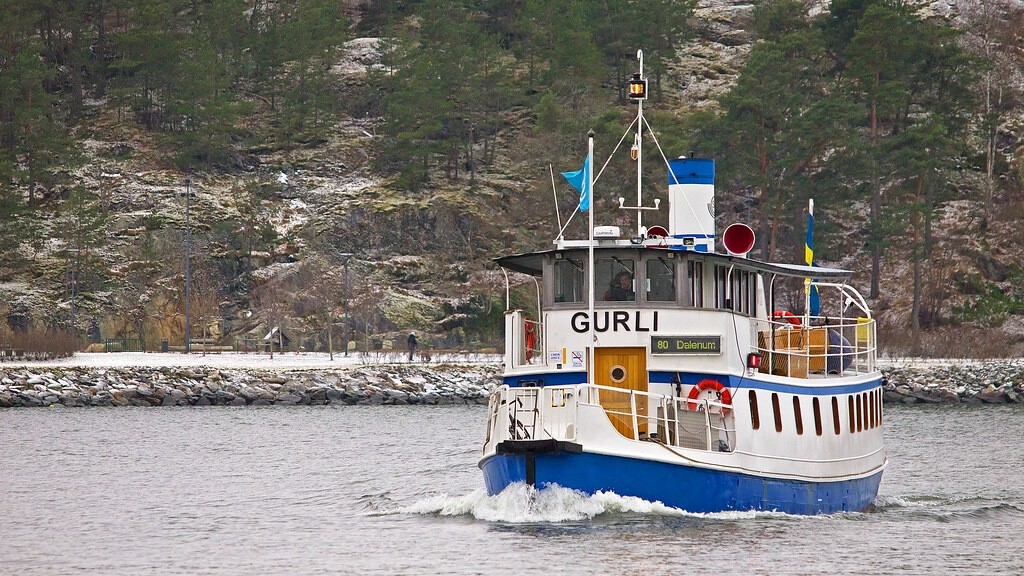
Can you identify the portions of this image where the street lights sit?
[340,252,354,357]
[184,179,192,355]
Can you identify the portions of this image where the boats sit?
[477,49,890,520]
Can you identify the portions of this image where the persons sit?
[612,271,633,300]
[407,331,418,360]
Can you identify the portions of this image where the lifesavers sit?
[523,317,535,359]
[767,310,802,330]
[687,380,732,419]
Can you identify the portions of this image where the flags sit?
[561,154,593,215]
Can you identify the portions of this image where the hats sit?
[411,332,415,336]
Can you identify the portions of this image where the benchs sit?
[758,330,829,380]
